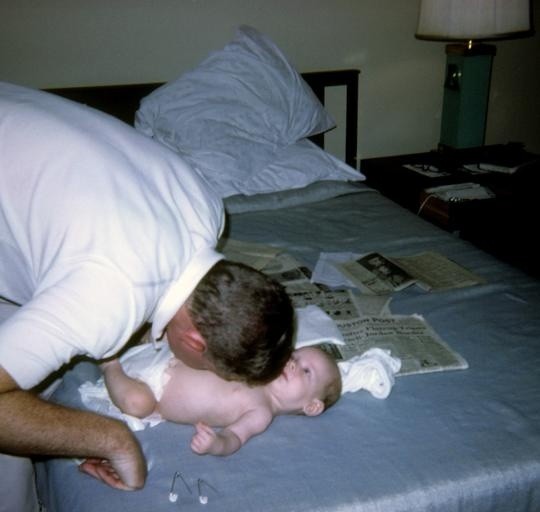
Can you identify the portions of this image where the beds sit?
[32,68,539,510]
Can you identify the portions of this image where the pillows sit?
[222,138,366,196]
[133,24,336,184]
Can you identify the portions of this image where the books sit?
[477,147,540,175]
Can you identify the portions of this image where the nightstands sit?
[358,141,540,269]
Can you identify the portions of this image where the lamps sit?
[413,0,532,149]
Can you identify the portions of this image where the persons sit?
[0,79,298,512]
[95,324,342,458]
